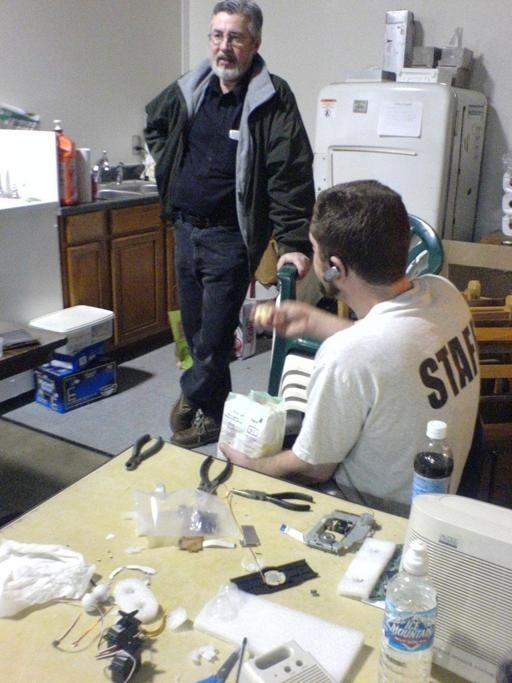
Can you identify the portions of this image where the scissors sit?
[195,647,242,683]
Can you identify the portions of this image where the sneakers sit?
[171,413,221,450]
[169,392,198,434]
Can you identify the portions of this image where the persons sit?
[141,1,315,449]
[220,180,481,517]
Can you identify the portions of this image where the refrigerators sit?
[312,80,488,243]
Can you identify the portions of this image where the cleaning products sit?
[52,119,78,206]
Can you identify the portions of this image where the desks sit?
[0,434,510,683]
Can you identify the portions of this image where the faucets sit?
[92,150,111,183]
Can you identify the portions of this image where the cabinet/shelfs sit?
[1,126,69,416]
[106,202,182,350]
[57,210,114,353]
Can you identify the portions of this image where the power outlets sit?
[130,133,144,157]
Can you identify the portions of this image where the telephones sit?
[233,640,338,683]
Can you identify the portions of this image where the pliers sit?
[196,455,232,494]
[124,434,163,471]
[232,488,313,511]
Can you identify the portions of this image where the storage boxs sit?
[226,294,259,361]
[46,342,106,371]
[32,358,120,417]
[27,303,118,352]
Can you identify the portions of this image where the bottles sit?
[377,540,439,681]
[412,418,455,499]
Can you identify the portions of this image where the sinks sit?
[95,189,143,200]
[107,179,158,194]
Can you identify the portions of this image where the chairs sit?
[264,404,497,520]
[262,207,445,421]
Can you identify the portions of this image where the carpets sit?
[1,330,283,461]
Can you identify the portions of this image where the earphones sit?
[322,265,341,282]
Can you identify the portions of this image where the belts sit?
[177,210,239,229]
